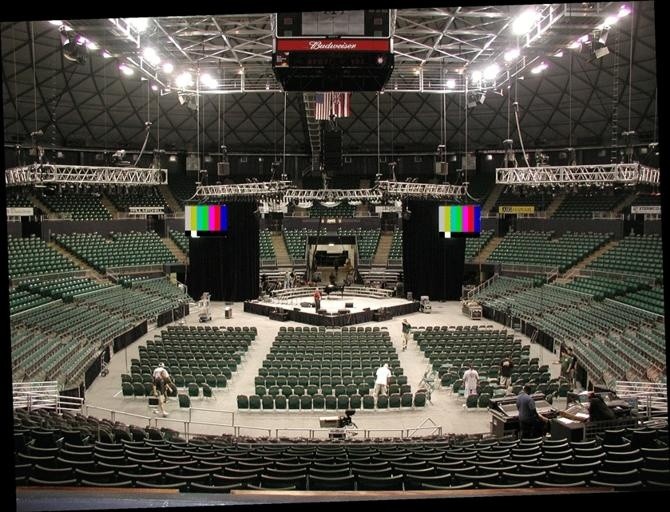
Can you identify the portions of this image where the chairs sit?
[2,110,670,494]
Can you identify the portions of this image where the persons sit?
[314,287,321,313]
[463,362,480,407]
[498,352,514,388]
[401,318,412,351]
[566,347,578,391]
[516,383,550,439]
[552,349,573,392]
[153,361,174,403]
[585,393,617,420]
[373,362,392,412]
[152,370,173,417]
[273,259,342,290]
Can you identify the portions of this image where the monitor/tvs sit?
[184,205,229,237]
[436,204,481,239]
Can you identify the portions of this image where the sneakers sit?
[151,408,169,418]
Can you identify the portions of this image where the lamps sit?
[1,1,670,210]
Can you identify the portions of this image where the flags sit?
[315,92,331,120]
[331,91,350,118]
